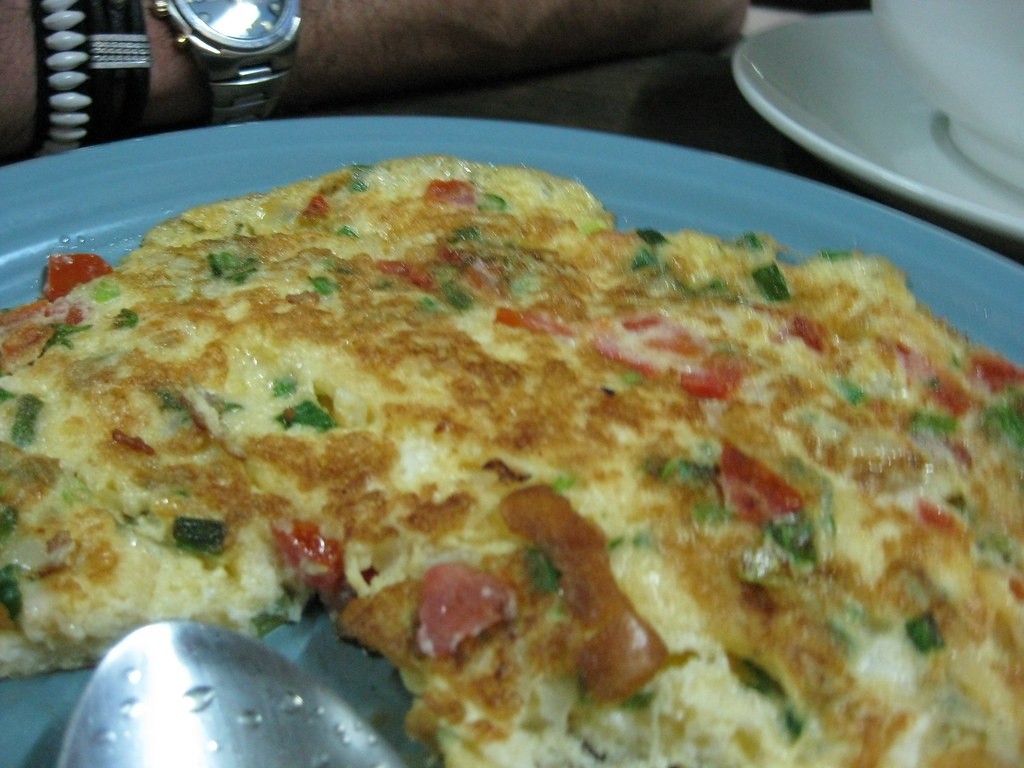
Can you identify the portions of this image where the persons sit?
[0,0,748,168]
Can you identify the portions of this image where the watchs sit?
[153,0,303,126]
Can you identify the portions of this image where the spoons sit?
[56,619,408,768]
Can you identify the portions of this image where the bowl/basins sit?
[870,0,1024,194]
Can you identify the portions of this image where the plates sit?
[730,10,1024,239]
[3,118,1024,768]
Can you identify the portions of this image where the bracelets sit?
[38,0,93,156]
[85,0,152,146]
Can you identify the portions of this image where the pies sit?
[1,157,1022,767]
